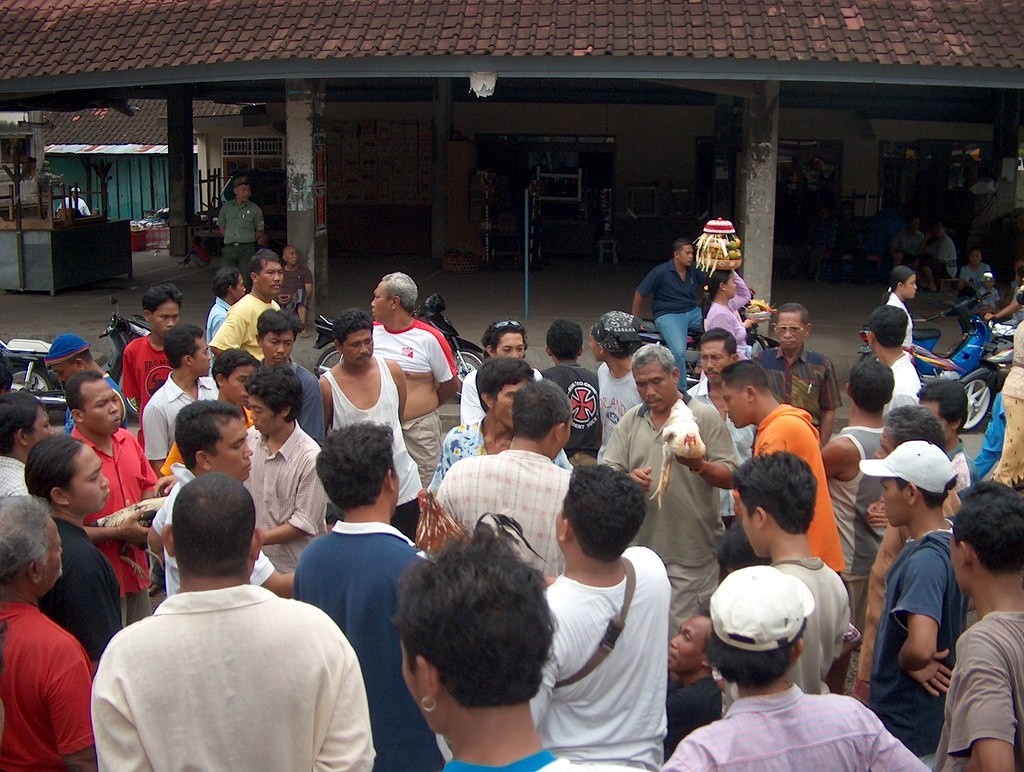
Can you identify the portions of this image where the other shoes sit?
[147,580,162,597]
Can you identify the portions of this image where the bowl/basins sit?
[745,312,771,320]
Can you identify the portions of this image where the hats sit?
[44,334,90,367]
[234,177,251,187]
[592,310,643,355]
[710,566,816,652]
[859,441,958,492]
[71,188,80,192]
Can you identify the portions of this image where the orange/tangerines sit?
[729,241,741,259]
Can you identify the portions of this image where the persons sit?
[604,344,740,682]
[294,422,446,772]
[859,441,975,772]
[42,207,1023,597]
[217,175,266,275]
[663,608,722,766]
[0,391,54,496]
[91,474,377,772]
[396,523,657,772]
[530,464,669,772]
[0,496,99,772]
[659,563,934,772]
[716,522,862,694]
[930,481,1024,772]
[24,436,123,673]
[56,186,100,215]
[734,451,850,691]
[0,351,14,395]
[65,370,162,628]
[854,406,960,705]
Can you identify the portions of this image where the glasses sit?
[490,321,521,333]
[774,325,803,336]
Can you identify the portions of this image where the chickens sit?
[413,488,547,561]
[87,494,169,579]
[648,398,706,509]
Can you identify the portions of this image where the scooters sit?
[313,292,484,402]
[0,295,152,425]
[637,318,782,390]
[857,290,1023,435]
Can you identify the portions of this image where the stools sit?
[599,239,618,266]
[939,278,961,292]
